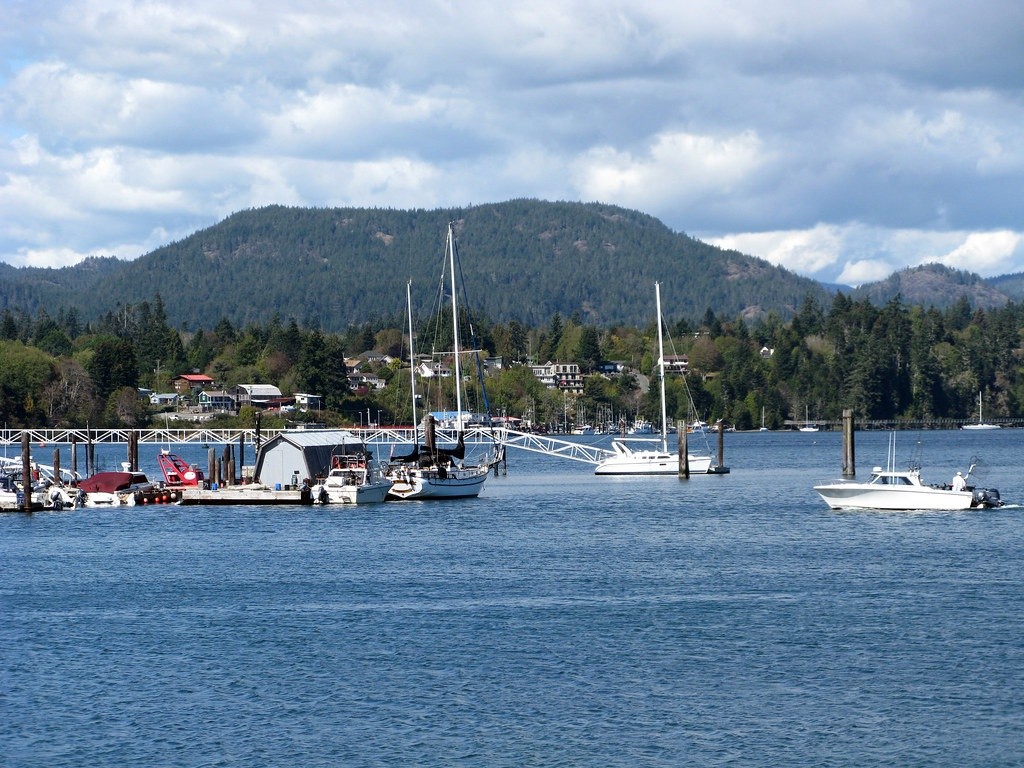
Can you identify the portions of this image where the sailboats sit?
[384,281,490,501]
[593,282,714,476]
[798,406,820,433]
[962,392,1003,431]
[758,406,768,432]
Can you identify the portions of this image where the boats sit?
[310,460,392,504]
[77,462,153,509]
[814,431,998,511]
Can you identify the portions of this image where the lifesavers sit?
[438,467,447,478]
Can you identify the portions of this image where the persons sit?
[953,472,966,492]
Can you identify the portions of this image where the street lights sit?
[377,408,382,429]
[359,412,364,428]
